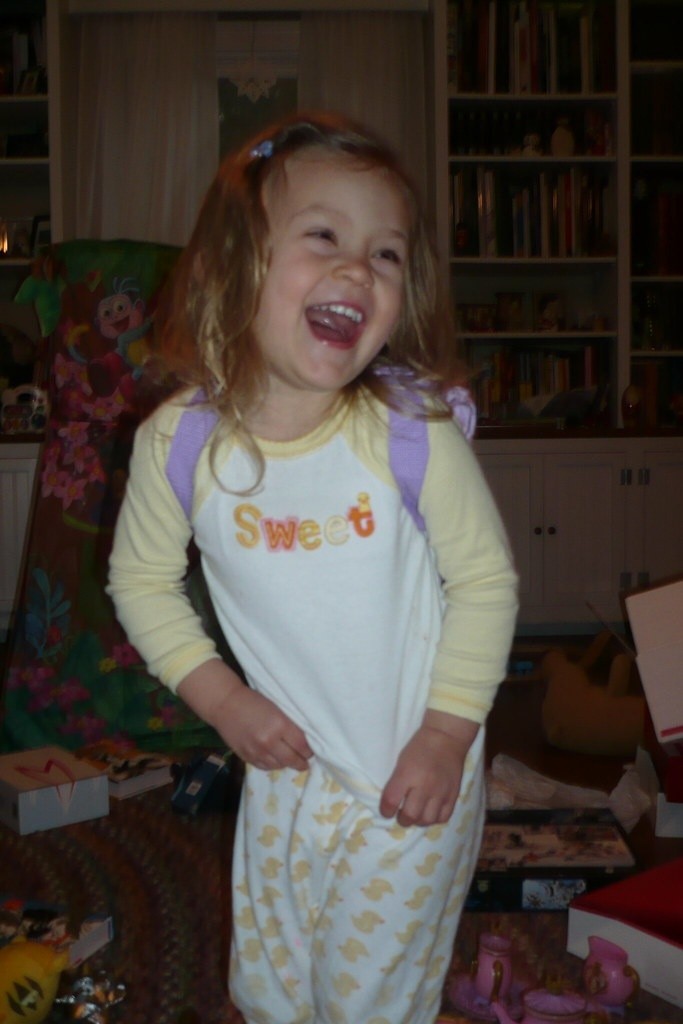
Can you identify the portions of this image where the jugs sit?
[583,936,640,1006]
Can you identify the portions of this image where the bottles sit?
[476,918,512,1018]
[520,967,588,1024]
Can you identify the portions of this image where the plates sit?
[448,968,527,1023]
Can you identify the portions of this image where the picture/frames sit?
[29,213,51,257]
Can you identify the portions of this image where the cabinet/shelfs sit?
[0,0,79,629]
[421,0,683,627]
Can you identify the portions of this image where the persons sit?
[102,111,520,1024]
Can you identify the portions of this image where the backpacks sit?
[163,356,479,526]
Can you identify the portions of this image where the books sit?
[464,344,599,419]
[447,0,615,94]
[450,165,604,258]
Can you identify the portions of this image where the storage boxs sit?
[0,741,229,835]
[626,574,683,744]
[564,859,683,1007]
[466,806,635,912]
